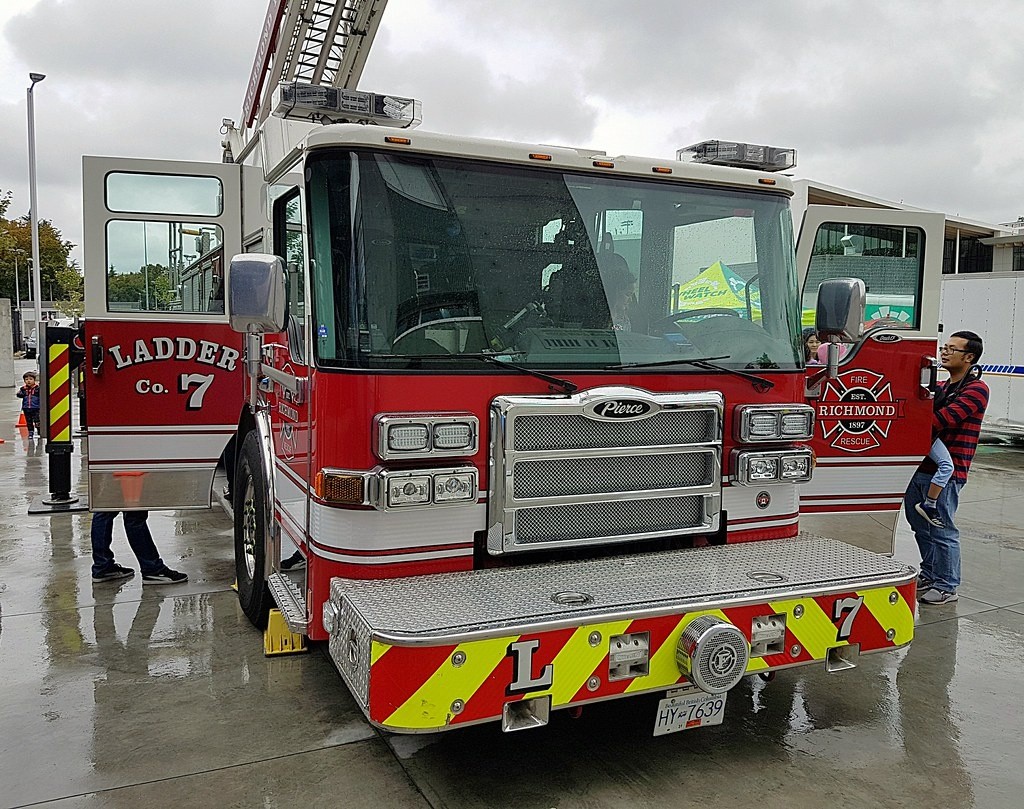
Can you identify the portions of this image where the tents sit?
[671,262,816,325]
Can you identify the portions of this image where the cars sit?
[25,329,38,359]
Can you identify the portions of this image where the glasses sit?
[938,345,969,357]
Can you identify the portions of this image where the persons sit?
[905,330,989,605]
[802,328,822,365]
[17,372,40,439]
[581,269,637,332]
[91,511,188,584]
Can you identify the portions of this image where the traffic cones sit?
[16,414,29,428]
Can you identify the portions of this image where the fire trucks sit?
[26,0,945,739]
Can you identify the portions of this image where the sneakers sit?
[915,500,947,529]
[92,564,135,583]
[28,433,34,439]
[921,587,960,605]
[916,574,934,591]
[142,567,188,584]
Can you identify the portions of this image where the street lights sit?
[26,258,33,302]
[47,279,54,301]
[184,254,197,265]
[152,280,158,309]
[138,300,141,309]
[9,248,24,311]
[27,72,47,331]
[138,292,142,311]
